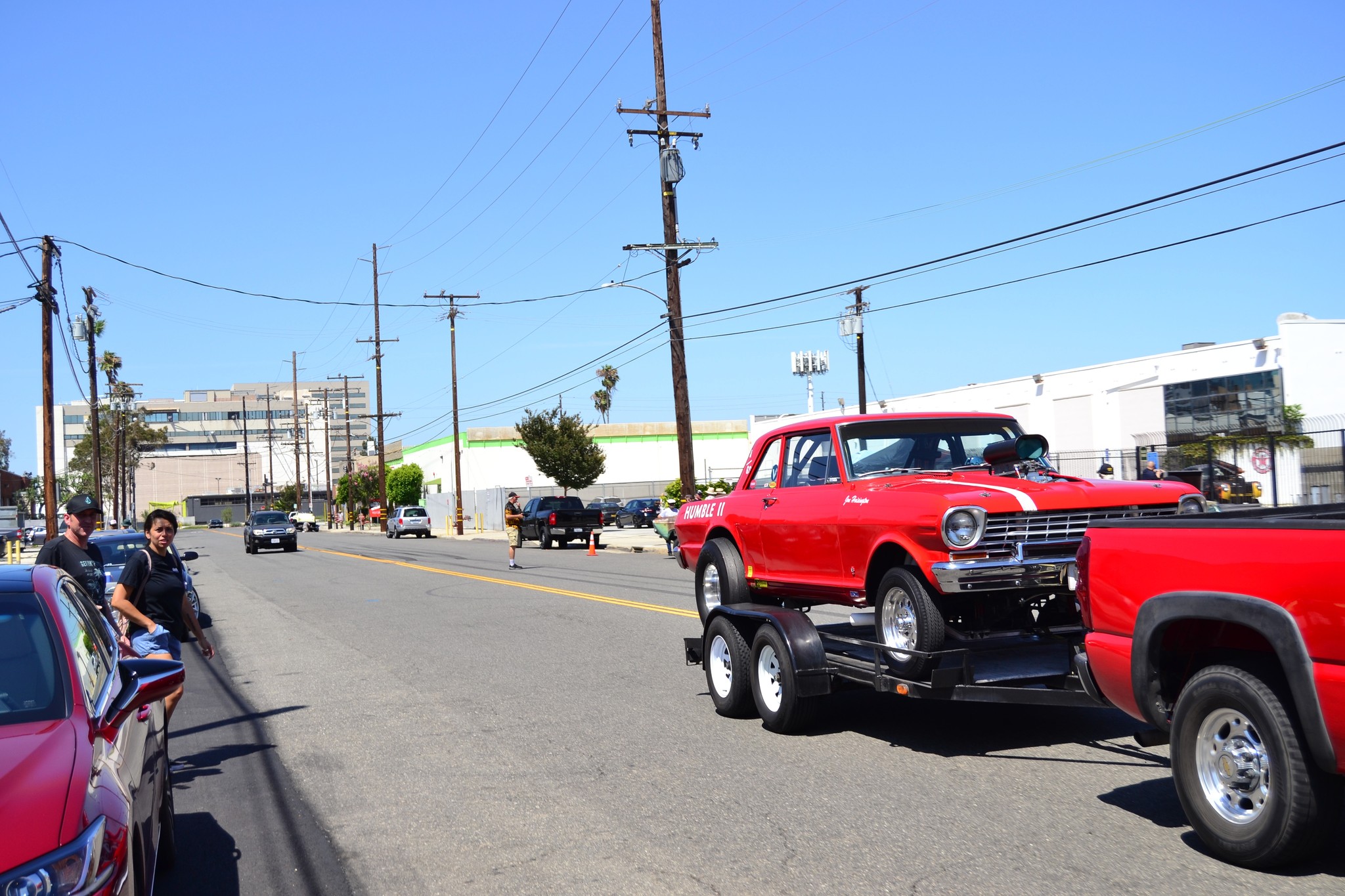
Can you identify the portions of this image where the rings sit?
[205,649,208,652]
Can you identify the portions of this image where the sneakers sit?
[668,551,672,556]
[509,563,522,569]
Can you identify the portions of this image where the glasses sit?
[686,499,690,501]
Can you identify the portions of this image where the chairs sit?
[414,512,420,516]
[0,615,50,711]
[409,514,414,516]
[804,455,841,487]
[256,518,264,525]
[119,549,137,564]
[100,545,123,565]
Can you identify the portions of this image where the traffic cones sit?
[586,531,598,555]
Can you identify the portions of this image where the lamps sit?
[1252,338,1268,350]
[837,397,845,408]
[1033,373,1044,385]
[440,456,443,459]
[879,399,888,408]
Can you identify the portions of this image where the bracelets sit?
[198,637,206,641]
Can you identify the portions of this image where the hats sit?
[66,494,104,514]
[697,490,703,493]
[509,492,520,498]
[715,488,726,494]
[121,519,132,526]
[1097,464,1114,475]
[705,487,717,494]
[338,510,342,512]
[666,499,676,503]
[110,520,117,525]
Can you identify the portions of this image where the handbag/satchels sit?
[117,608,132,646]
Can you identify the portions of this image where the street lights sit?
[215,478,221,493]
[346,419,388,532]
[601,281,696,498]
[237,479,251,516]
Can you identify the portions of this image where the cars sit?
[585,502,622,526]
[674,411,1224,681]
[24,526,48,545]
[57,518,201,631]
[208,519,223,528]
[0,563,189,896]
[290,509,316,527]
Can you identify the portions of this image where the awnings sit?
[423,478,441,486]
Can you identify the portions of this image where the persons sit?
[1142,461,1164,481]
[1097,464,1114,480]
[504,491,524,570]
[25,528,34,541]
[692,490,704,502]
[704,487,717,500]
[32,493,130,654]
[119,519,132,530]
[684,494,692,503]
[302,522,310,533]
[657,499,680,556]
[338,510,343,529]
[108,508,215,772]
[714,488,727,496]
[109,519,117,530]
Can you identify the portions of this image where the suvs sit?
[243,509,297,555]
[386,505,432,539]
[615,498,661,529]
[590,496,625,508]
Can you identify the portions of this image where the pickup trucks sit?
[288,511,320,532]
[1059,500,1345,870]
[519,496,604,549]
